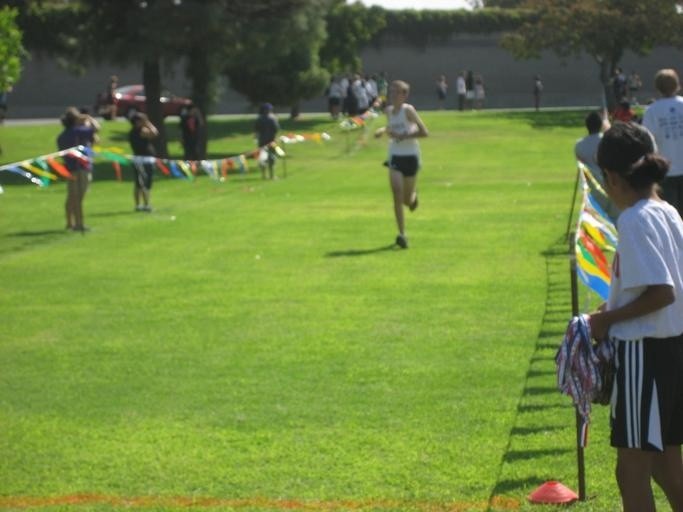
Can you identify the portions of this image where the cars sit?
[92,84,196,121]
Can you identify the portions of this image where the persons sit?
[534,72,544,111]
[56,105,102,231]
[588,125,683,512]
[323,70,381,119]
[255,102,278,180]
[576,112,607,169]
[1,86,12,118]
[642,68,683,205]
[181,106,208,160]
[128,110,159,213]
[435,68,485,110]
[107,74,118,119]
[374,81,429,248]
[613,66,643,123]
